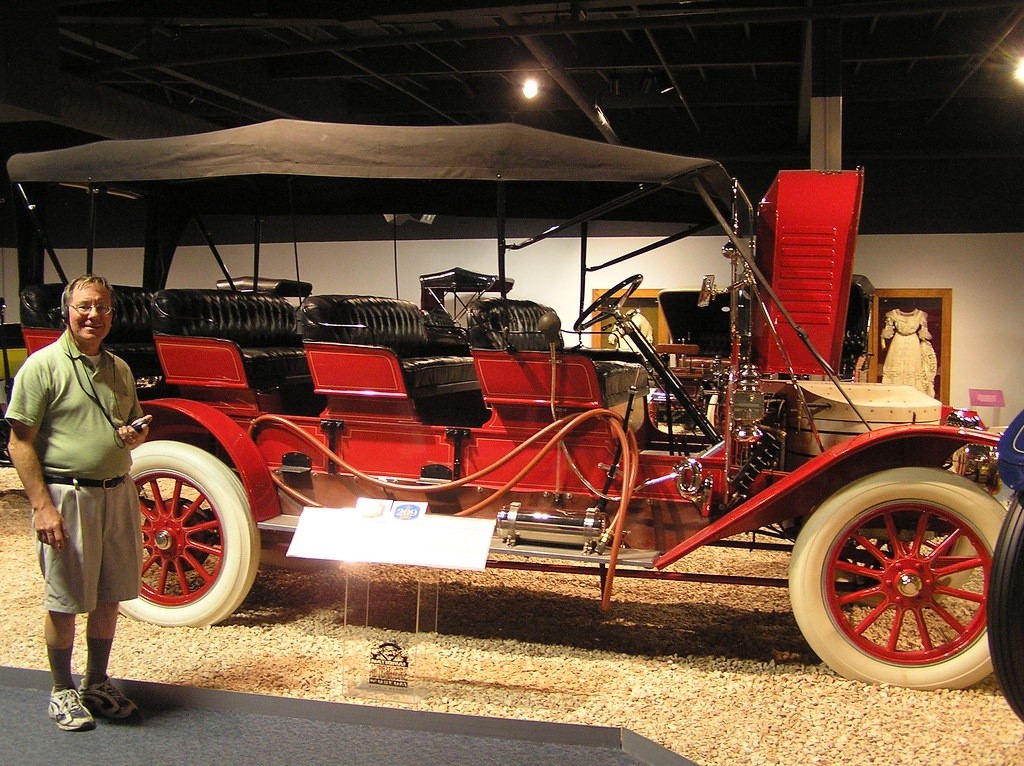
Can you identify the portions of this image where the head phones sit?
[61,289,117,324]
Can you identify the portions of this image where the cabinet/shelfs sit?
[592,288,952,407]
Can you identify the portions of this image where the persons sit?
[4,278,152,731]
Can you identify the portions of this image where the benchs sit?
[300,295,482,399]
[18,281,162,376]
[466,298,650,405]
[150,289,314,389]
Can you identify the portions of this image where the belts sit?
[43,474,128,489]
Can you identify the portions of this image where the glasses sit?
[72,302,115,316]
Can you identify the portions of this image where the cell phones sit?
[132,419,148,432]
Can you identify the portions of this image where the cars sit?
[5,114,1011,694]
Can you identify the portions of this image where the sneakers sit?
[78,674,139,719]
[47,685,96,731]
